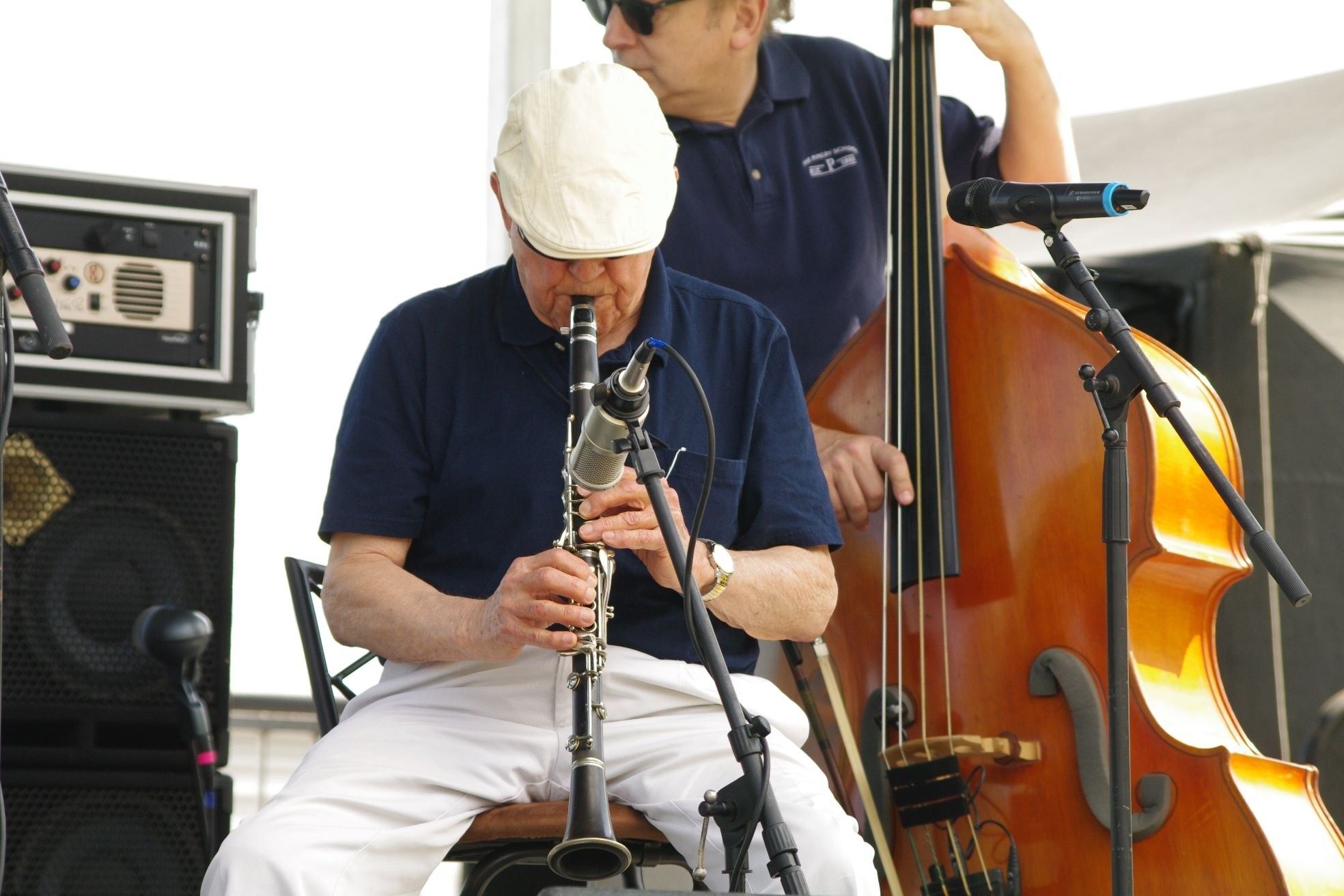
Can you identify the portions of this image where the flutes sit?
[548,295,630,882]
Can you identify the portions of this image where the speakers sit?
[7,764,232,894]
[1,397,227,766]
[1099,243,1343,831]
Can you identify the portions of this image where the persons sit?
[202,63,884,896]
[583,0,1080,687]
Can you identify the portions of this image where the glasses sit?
[581,0,685,36]
[516,224,628,261]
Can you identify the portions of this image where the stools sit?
[445,802,708,896]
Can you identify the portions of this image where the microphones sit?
[128,606,213,663]
[567,336,654,490]
[946,178,1152,228]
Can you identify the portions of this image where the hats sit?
[493,61,680,259]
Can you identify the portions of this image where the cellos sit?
[771,0,1343,896]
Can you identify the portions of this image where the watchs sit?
[679,538,734,602]
